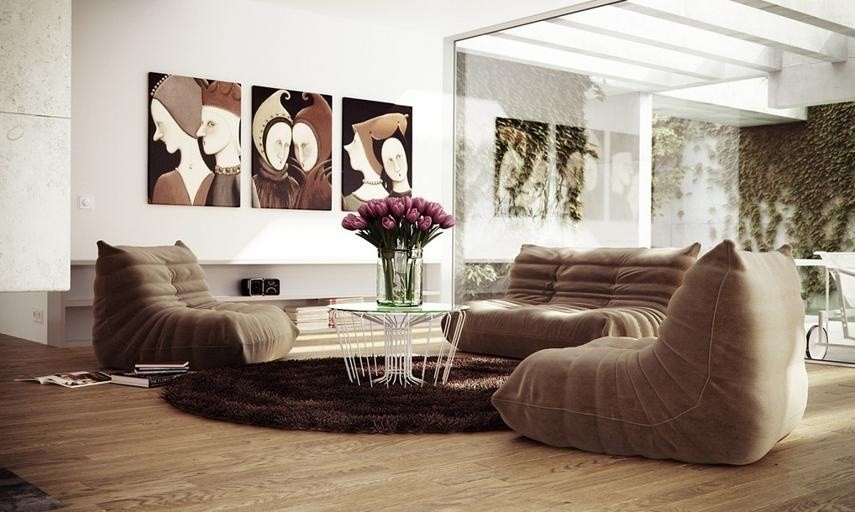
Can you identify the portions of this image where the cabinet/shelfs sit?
[49,251,453,347]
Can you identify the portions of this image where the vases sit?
[376,247,424,308]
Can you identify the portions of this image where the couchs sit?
[443,243,702,360]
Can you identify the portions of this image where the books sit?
[110,360,190,388]
[12,370,111,388]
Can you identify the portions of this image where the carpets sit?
[162,353,523,434]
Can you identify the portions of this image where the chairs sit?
[91,239,301,366]
[491,239,809,466]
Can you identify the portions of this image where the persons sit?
[252,90,300,208]
[369,121,412,198]
[196,81,241,207]
[151,75,215,206]
[292,92,332,210]
[343,113,410,212]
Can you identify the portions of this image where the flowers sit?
[342,195,456,248]
